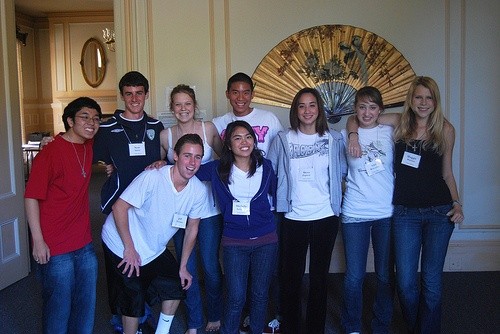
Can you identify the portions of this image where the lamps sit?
[102,28,116,53]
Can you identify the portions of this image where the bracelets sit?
[348,132,359,135]
[452,200,463,208]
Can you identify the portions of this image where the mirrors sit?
[80,37,106,88]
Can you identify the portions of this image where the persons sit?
[159,85,224,333]
[89,71,166,222]
[340,85,395,334]
[145,121,278,334]
[24,96,113,334]
[212,72,285,163]
[100,134,210,334]
[346,76,464,334]
[267,87,348,333]
